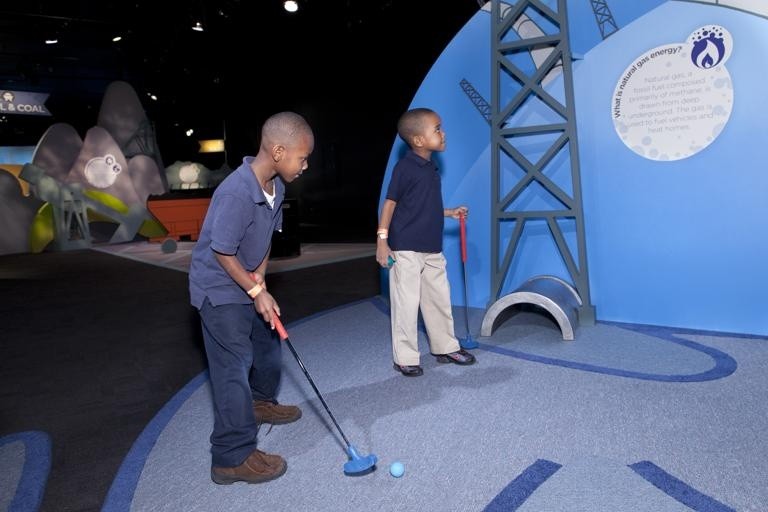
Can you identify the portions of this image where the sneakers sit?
[209,449,288,486]
[392,361,423,377]
[436,349,477,366]
[252,398,302,425]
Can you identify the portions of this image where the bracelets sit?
[248,284,263,300]
[377,227,390,240]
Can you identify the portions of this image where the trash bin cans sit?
[269,198,301,260]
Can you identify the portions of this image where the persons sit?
[188,111,316,485]
[374,106,477,378]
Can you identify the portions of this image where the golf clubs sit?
[247,271,377,473]
[460,209,479,349]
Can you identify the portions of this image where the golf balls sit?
[388,256,393,266]
[390,462,404,477]
